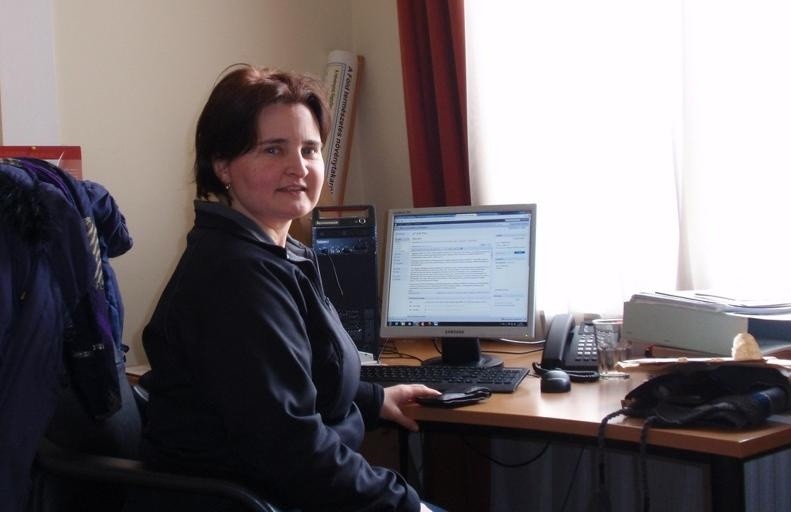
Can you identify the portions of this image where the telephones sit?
[532,312,614,382]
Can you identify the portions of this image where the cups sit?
[593,318,632,382]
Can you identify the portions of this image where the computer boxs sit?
[310,205,380,363]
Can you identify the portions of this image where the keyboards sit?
[361,365,528,393]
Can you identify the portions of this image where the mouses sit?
[540,369,571,393]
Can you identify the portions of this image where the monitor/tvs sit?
[379,204,537,367]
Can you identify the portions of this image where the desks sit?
[125,335,791,512]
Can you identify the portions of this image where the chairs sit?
[28,454,278,512]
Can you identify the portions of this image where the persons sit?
[141,63,442,511]
